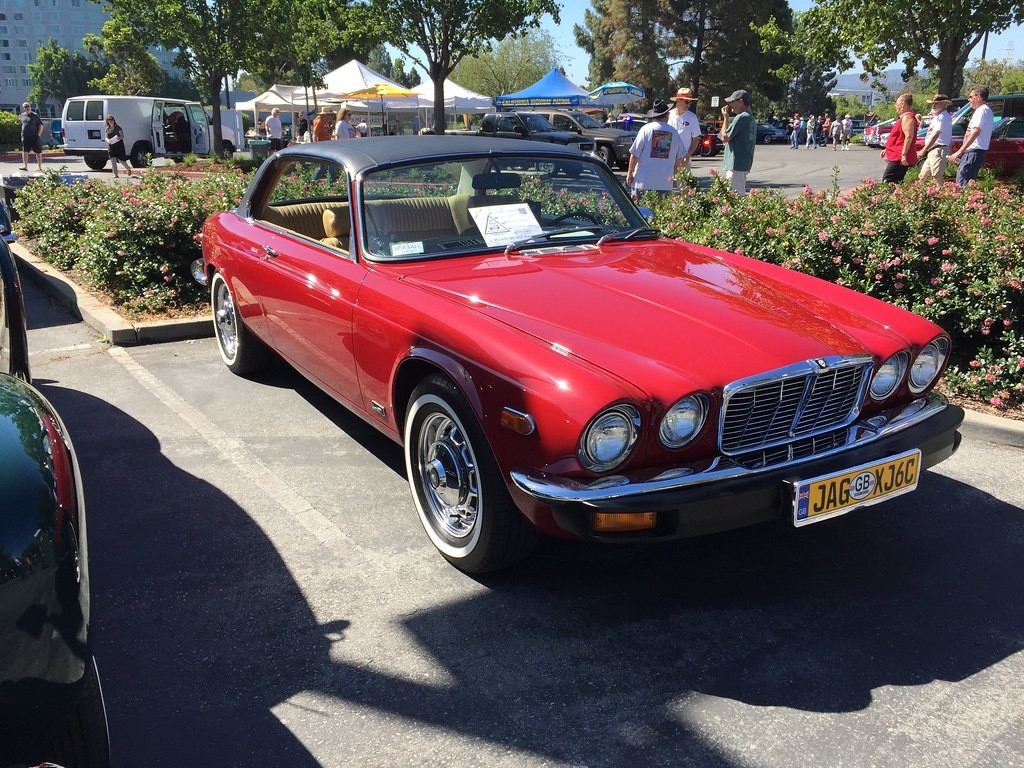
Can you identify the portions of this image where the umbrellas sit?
[587,81,645,105]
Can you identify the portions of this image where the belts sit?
[928,145,946,152]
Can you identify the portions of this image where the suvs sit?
[509,108,639,171]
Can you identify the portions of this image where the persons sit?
[19,101,44,171]
[720,90,757,196]
[335,108,355,139]
[947,86,993,186]
[312,107,337,188]
[881,94,918,183]
[356,117,368,137]
[667,88,702,189]
[295,111,308,143]
[626,99,687,207]
[916,94,952,183]
[265,108,282,156]
[104,115,132,178]
[766,108,926,151]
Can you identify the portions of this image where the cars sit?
[602,90,1023,180]
[0,192,112,768]
[202,133,965,577]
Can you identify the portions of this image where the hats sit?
[647,100,674,117]
[724,90,749,103]
[670,88,699,101]
[317,107,336,115]
[298,111,304,116]
[795,114,799,117]
[926,94,953,105]
[845,114,851,118]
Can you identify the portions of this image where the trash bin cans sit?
[248,139,272,161]
[282,135,297,147]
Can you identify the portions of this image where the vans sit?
[61,94,236,170]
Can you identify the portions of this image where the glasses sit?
[969,94,982,99]
[108,119,114,120]
[24,106,29,108]
[685,101,692,104]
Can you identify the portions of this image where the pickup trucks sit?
[418,112,596,178]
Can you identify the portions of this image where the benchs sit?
[259,188,472,251]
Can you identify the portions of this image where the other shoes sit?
[112,175,118,178]
[790,144,850,150]
[128,167,131,175]
[19,166,28,171]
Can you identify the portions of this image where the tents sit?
[234,58,613,139]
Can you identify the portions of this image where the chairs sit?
[320,206,370,252]
